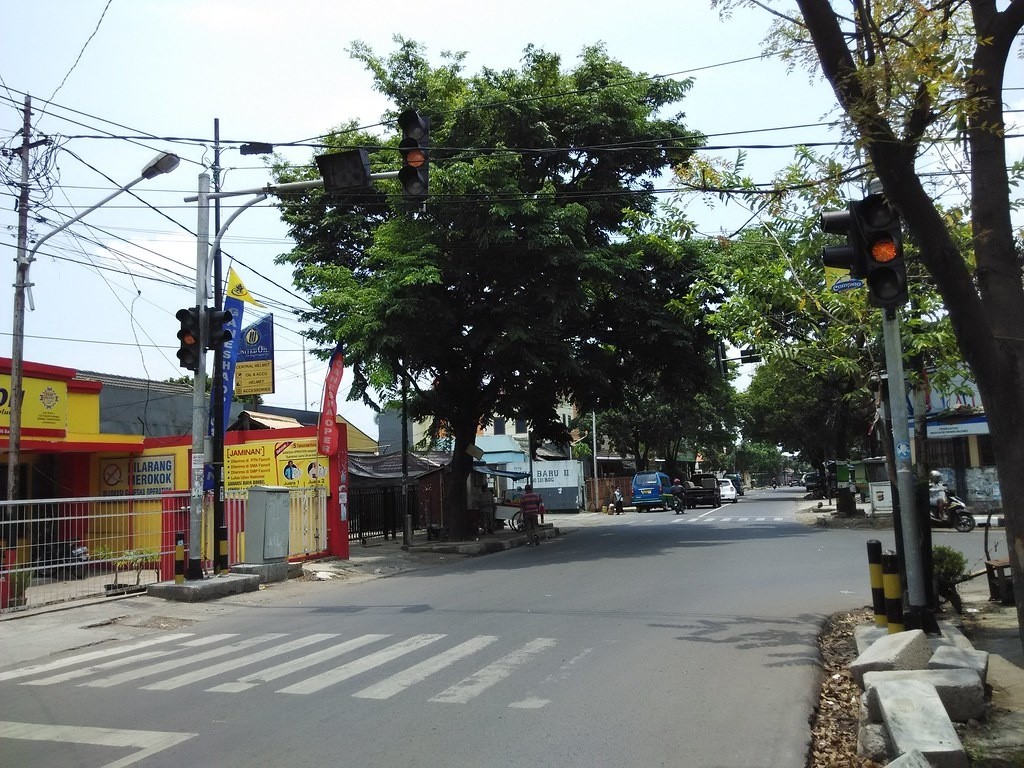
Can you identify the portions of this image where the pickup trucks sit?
[683,473,722,509]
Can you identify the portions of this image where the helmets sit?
[929,470,944,482]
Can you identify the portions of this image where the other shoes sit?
[525,535,540,546]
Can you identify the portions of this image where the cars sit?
[716,479,738,503]
[773,469,828,497]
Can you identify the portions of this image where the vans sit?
[631,471,675,512]
[723,472,744,495]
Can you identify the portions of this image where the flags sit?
[207,295,244,437]
[316,337,344,454]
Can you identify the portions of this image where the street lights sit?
[23,147,178,314]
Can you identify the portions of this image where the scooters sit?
[672,489,686,515]
[928,487,975,532]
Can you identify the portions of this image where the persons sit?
[518,484,541,547]
[771,476,778,488]
[670,478,687,510]
[613,486,625,516]
[929,470,956,521]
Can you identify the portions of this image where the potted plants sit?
[9,562,35,609]
[122,548,160,594]
[91,546,132,596]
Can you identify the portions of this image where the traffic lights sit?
[815,197,862,280]
[175,309,199,368]
[396,109,431,199]
[860,192,912,307]
[314,147,372,196]
[204,305,234,351]
